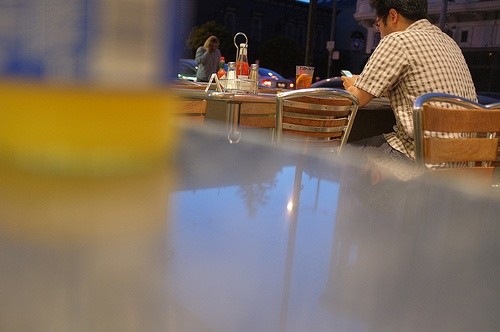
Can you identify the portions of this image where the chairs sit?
[413,93,500,187]
[276,87,360,160]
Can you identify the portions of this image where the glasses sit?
[372,14,386,32]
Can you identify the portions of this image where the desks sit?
[203,91,396,141]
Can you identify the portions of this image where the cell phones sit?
[340,70,352,77]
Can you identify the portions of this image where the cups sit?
[296,66,314,89]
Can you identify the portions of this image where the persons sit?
[195,36,222,82]
[341,0,479,171]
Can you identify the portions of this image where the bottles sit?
[217,57,226,92]
[250,63,259,95]
[236,43,250,77]
[226,61,237,89]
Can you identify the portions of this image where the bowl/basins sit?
[216,78,256,96]
